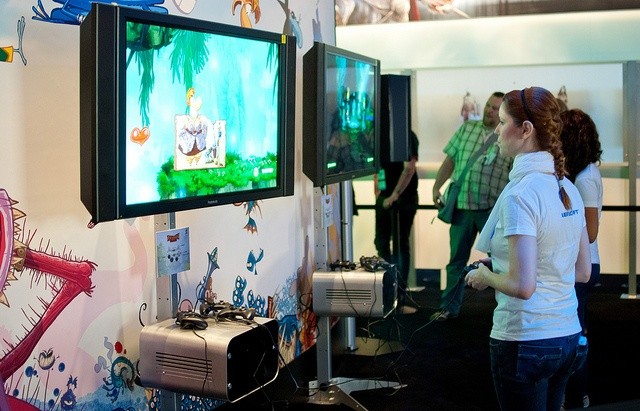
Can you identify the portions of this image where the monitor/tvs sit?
[303,42,380,188]
[381,74,411,163]
[80,3,296,224]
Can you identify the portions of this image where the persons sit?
[558,108,604,411]
[373,130,419,313]
[464,87,593,411]
[428,91,516,323]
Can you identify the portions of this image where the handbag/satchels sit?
[436,134,499,223]
